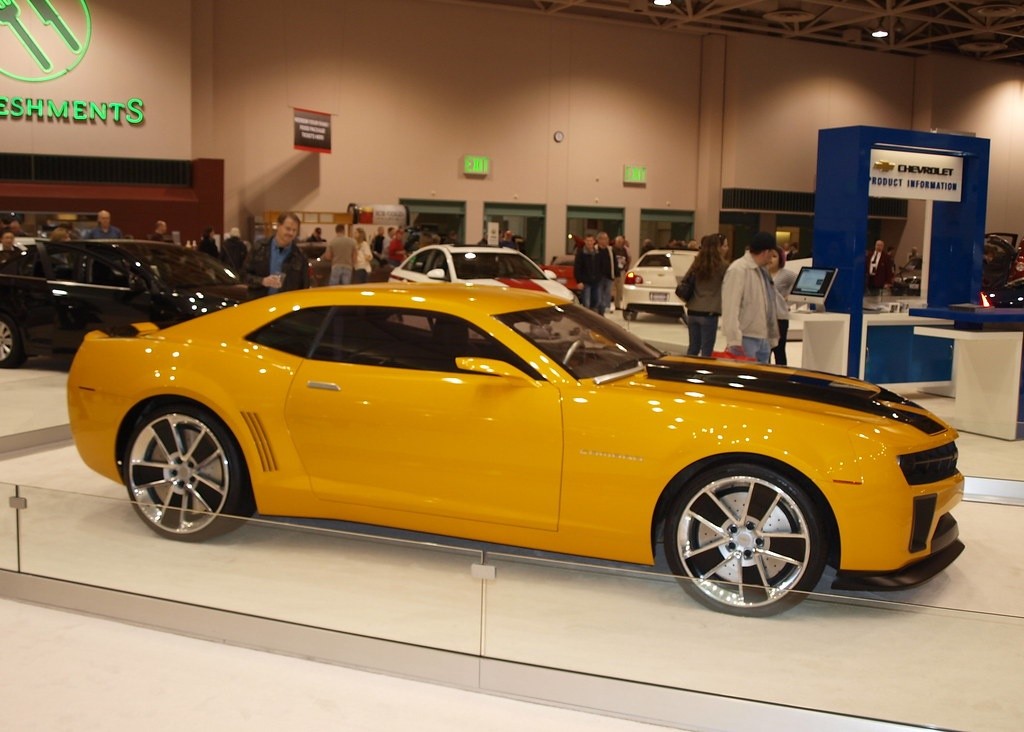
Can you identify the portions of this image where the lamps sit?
[871,16,889,37]
[893,17,905,31]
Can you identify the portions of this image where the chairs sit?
[91,261,118,287]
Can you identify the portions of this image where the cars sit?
[387,243,586,343]
[980,230,1024,308]
[890,256,923,297]
[0,235,319,369]
[296,239,400,287]
[617,249,701,321]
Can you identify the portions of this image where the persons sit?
[49,222,81,243]
[306,228,326,258]
[239,212,311,298]
[612,235,632,311]
[476,228,531,275]
[351,227,373,283]
[887,246,896,284]
[763,244,799,368]
[720,231,780,367]
[149,220,168,242]
[866,240,892,297]
[323,225,357,285]
[573,233,597,315]
[593,231,622,317]
[373,224,463,276]
[0,217,28,266]
[218,226,247,273]
[90,209,134,240]
[681,233,730,357]
[640,238,656,258]
[786,242,804,260]
[668,239,697,249]
[196,227,221,259]
[909,246,921,266]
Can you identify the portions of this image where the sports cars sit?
[67,281,967,619]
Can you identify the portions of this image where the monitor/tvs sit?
[788,265,838,312]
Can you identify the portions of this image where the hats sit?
[752,231,781,251]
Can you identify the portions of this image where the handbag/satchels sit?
[675,283,692,302]
[365,260,372,273]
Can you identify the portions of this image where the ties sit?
[872,252,878,263]
